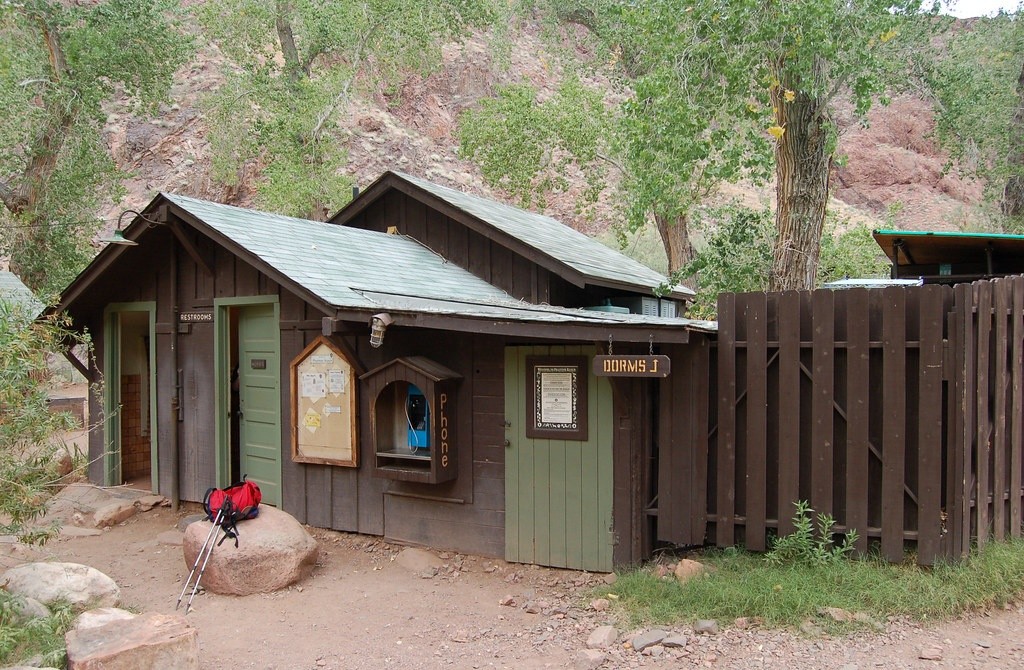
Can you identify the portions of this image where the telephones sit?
[404,386,431,447]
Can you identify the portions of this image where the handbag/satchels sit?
[202,473,262,548]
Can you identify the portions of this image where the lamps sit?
[96,209,167,245]
[369,314,393,348]
